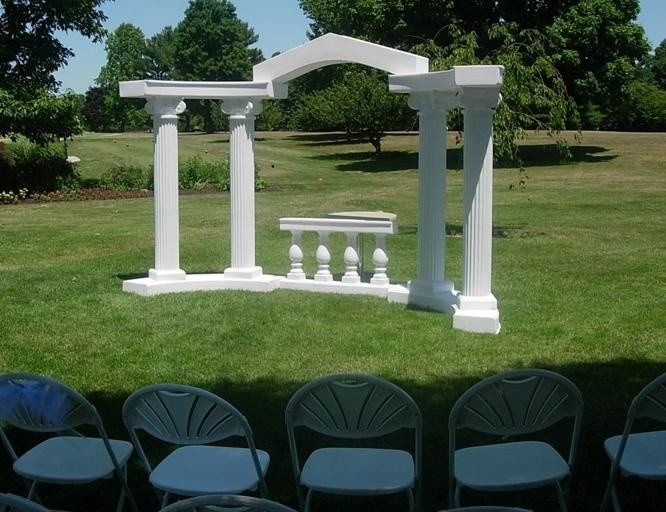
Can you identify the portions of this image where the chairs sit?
[159,493,295,512]
[441,504,534,512]
[0,373,133,512]
[447,365,584,512]
[121,383,271,512]
[602,372,666,512]
[283,372,424,512]
[0,492,49,512]
